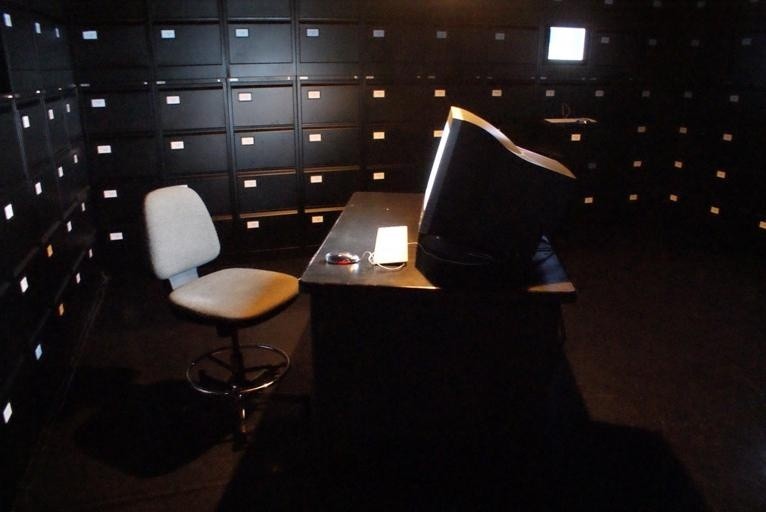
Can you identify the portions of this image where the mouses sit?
[323,251,359,266]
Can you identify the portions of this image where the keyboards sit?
[371,224,409,269]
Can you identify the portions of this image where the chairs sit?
[140,180,299,443]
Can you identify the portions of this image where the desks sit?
[300,191,577,461]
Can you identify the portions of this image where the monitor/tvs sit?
[544,22,591,68]
[417,105,577,264]
